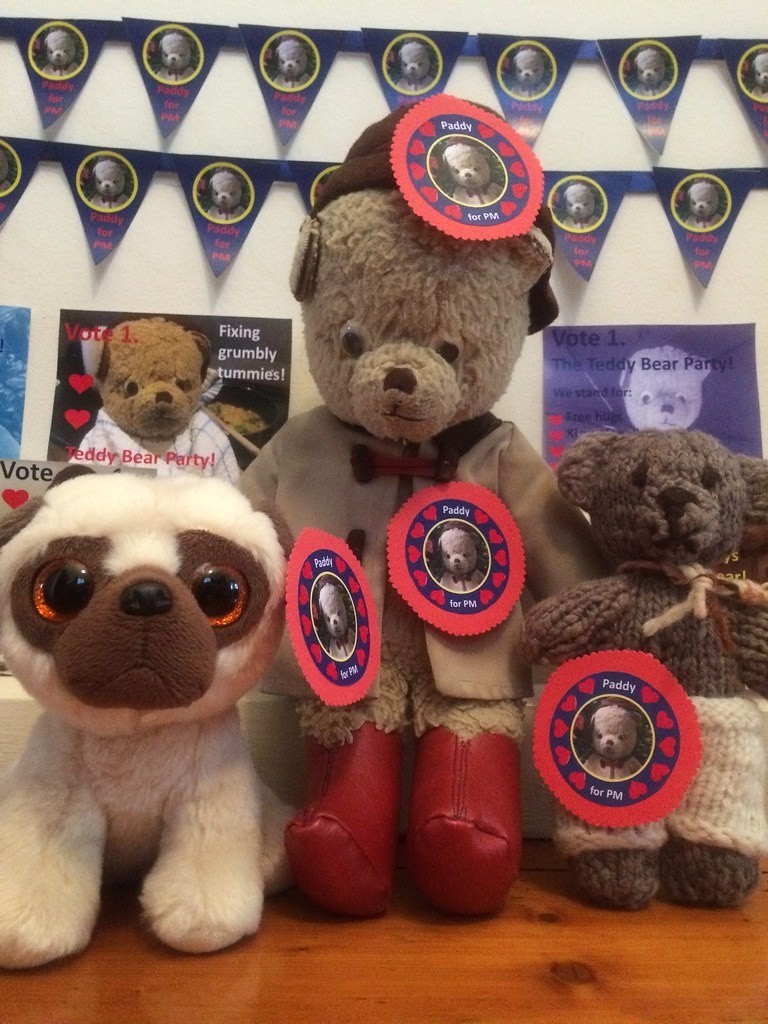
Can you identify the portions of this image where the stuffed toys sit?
[687,180,723,229]
[39,27,80,78]
[583,697,644,779]
[91,158,128,210]
[49,315,242,493]
[519,428,767,913]
[438,520,486,593]
[0,464,295,971]
[512,45,548,96]
[748,49,768,101]
[619,346,711,429]
[242,92,620,918]
[443,137,503,204]
[274,36,311,89]
[207,170,245,220]
[397,38,434,91]
[562,180,601,229]
[634,45,670,96]
[156,28,194,82]
[319,576,355,660]
